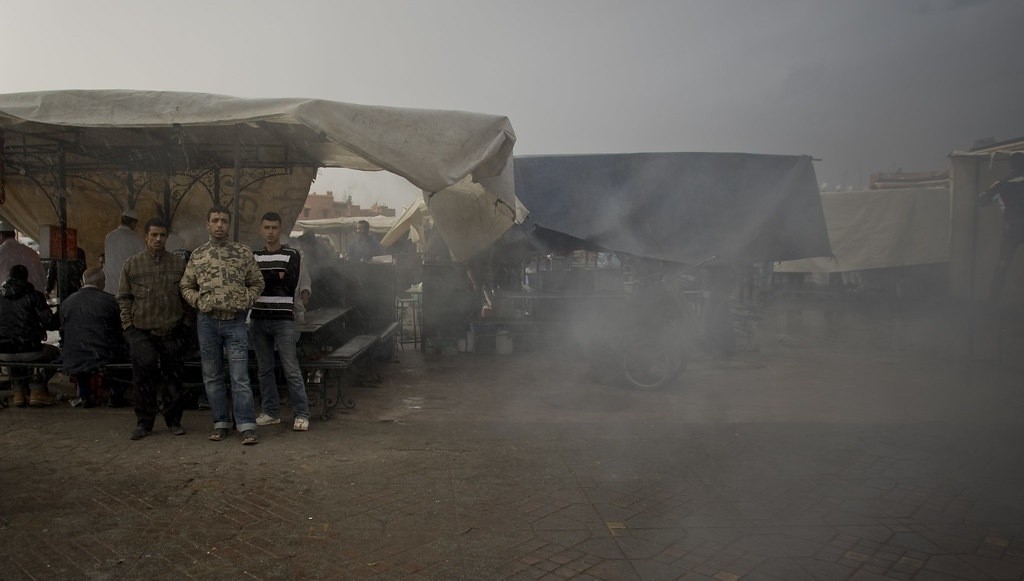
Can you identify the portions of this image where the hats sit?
[0,221,14,231]
[123,208,139,220]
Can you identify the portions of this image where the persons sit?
[975,152,1024,318]
[282,224,341,261]
[244,211,314,431]
[102,210,149,301]
[347,220,383,260]
[47,247,88,349]
[181,207,268,446]
[100,254,108,275]
[59,266,135,410]
[1,264,65,406]
[0,221,51,305]
[118,218,192,442]
[172,249,201,357]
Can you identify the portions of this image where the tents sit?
[0,92,533,305]
[508,154,835,271]
[772,183,954,271]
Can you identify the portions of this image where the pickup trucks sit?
[484,280,761,395]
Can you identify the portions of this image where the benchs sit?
[1,303,403,421]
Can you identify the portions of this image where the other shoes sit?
[208,429,227,440]
[30,385,55,405]
[170,424,185,435]
[14,387,27,406]
[242,430,259,443]
[130,426,148,440]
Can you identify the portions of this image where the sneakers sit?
[255,413,281,425]
[293,418,309,430]
